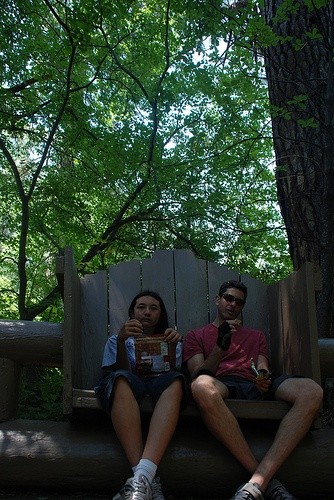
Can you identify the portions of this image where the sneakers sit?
[151,477,165,500]
[263,478,296,500]
[230,482,264,500]
[113,474,152,500]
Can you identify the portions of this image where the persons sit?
[94,291,186,500]
[183,280,323,500]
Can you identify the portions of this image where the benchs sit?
[56,246,323,431]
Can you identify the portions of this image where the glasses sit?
[222,294,246,306]
